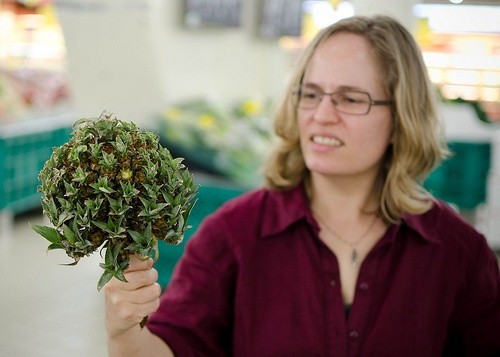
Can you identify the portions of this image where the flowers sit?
[31,110,201,330]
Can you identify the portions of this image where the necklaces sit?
[310,209,381,261]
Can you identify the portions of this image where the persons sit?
[103,15,500,357]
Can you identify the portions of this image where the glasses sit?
[290,84,396,115]
